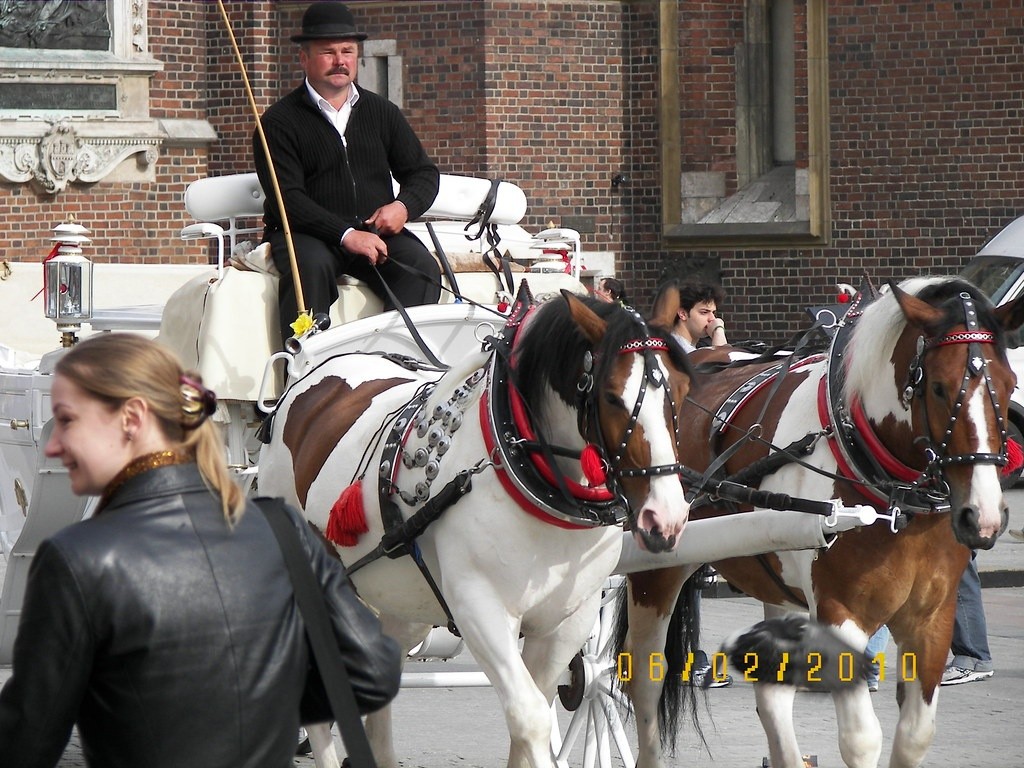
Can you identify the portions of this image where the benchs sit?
[180,172,547,275]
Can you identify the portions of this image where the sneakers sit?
[683,651,733,690]
[940,663,996,686]
[866,674,880,692]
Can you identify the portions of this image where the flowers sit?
[291,310,317,333]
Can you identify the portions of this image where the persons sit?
[597,277,623,303]
[0,328,401,768]
[669,282,733,689]
[860,547,994,690]
[252,1,440,389]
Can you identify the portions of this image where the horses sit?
[256,288,704,768]
[610,272,1024,768]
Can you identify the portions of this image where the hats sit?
[289,1,369,43]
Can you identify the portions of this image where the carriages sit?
[0,171,1024,768]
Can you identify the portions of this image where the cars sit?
[960,215,1024,490]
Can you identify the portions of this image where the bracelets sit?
[712,326,725,338]
